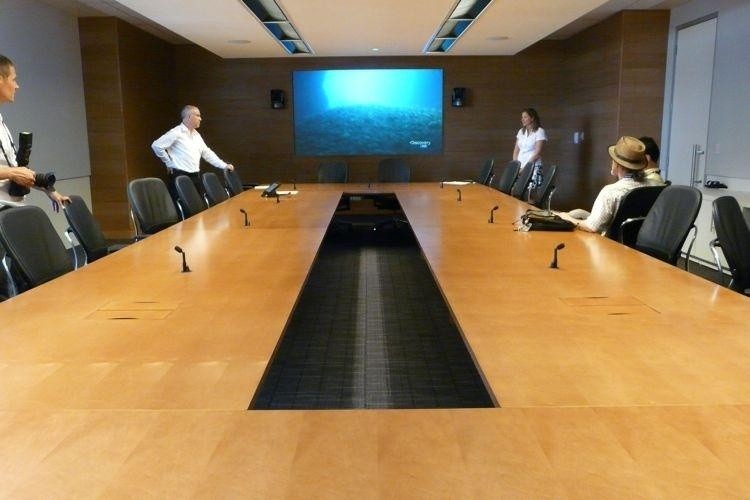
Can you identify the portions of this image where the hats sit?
[608,136,648,170]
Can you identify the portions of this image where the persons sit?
[149,101,235,193]
[511,106,546,202]
[549,136,666,233]
[0,51,74,299]
[635,136,665,184]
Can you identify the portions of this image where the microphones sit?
[491,206,499,223]
[442,181,443,188]
[551,243,565,268]
[240,209,248,226]
[275,192,280,202]
[174,245,190,272]
[368,178,371,187]
[457,189,462,201]
[294,178,297,190]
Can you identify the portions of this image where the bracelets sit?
[575,220,580,228]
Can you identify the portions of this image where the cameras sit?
[34,171,57,190]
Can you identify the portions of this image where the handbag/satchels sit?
[521,209,575,231]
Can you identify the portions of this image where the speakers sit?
[451,87,466,108]
[270,89,286,109]
